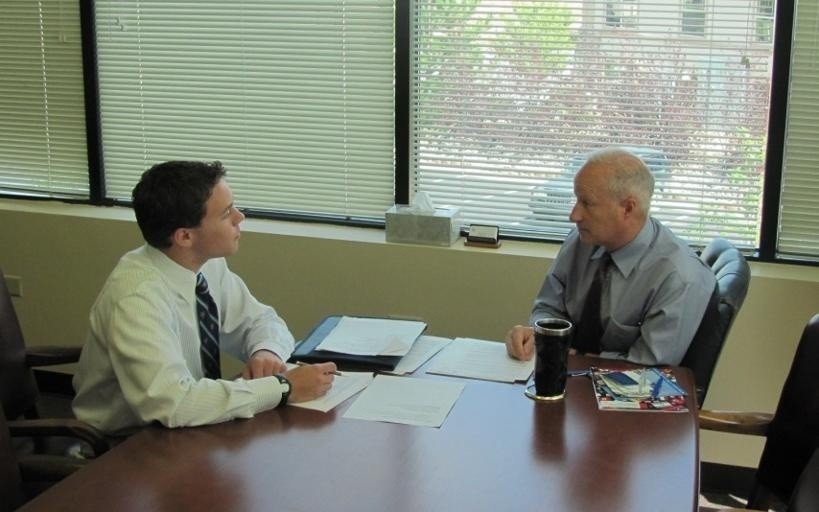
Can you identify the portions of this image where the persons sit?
[507,148,718,367]
[54,161,336,464]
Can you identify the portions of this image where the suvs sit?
[529,146,673,222]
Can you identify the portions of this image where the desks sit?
[17,339,700,512]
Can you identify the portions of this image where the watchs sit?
[272,373,291,410]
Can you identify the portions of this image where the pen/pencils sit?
[649,376,664,399]
[296,361,345,377]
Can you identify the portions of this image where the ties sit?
[579,253,615,354]
[194,273,223,380]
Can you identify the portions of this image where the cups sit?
[533,318,572,397]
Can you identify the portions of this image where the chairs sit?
[678,238,752,409]
[697,309,819,512]
[0,267,111,512]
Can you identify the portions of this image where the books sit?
[590,366,688,416]
